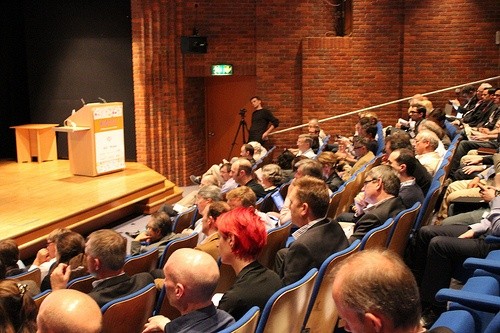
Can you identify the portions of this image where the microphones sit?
[98,97,106,103]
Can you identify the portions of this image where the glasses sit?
[410,111,419,115]
[364,178,377,187]
[492,94,500,98]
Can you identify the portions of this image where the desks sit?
[9,124,60,165]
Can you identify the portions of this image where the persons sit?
[331,248,427,333]
[130,213,176,256]
[182,83,500,286]
[0,226,153,333]
[163,249,236,333]
[142,206,283,333]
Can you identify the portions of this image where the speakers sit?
[182,35,207,54]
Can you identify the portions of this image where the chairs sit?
[4,113,500,333]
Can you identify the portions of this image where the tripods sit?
[226,120,249,161]
